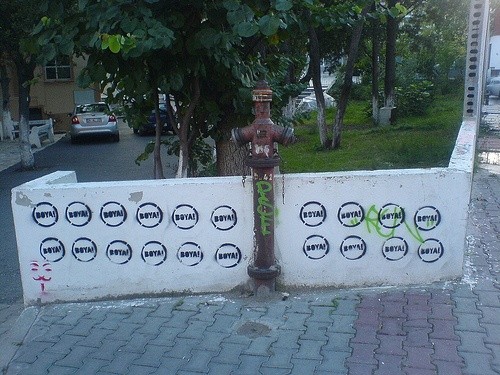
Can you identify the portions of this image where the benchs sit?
[10,118,55,149]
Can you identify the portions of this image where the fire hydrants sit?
[230,88,298,299]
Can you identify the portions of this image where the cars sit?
[133,103,178,135]
[67,102,119,144]
